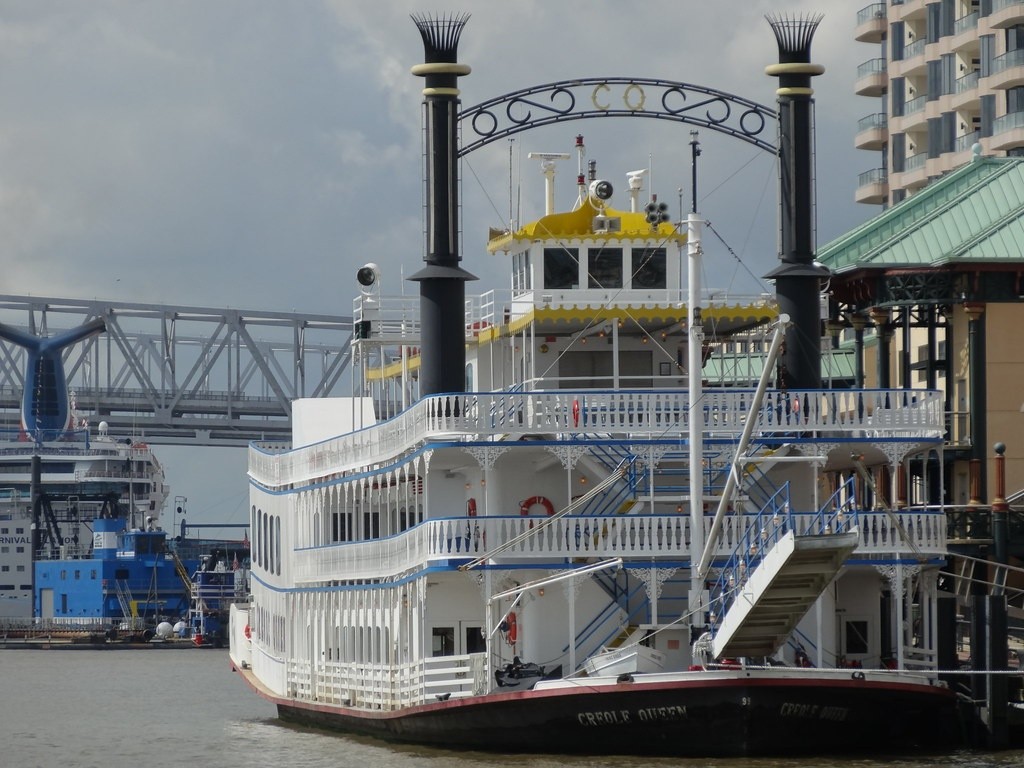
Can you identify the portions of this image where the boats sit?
[227,13,951,743]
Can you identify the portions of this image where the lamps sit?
[772,514,779,527]
[785,501,792,513]
[677,506,682,513]
[464,482,470,489]
[582,321,621,343]
[761,528,769,539]
[719,593,724,603]
[729,575,735,587]
[825,523,832,534]
[710,611,716,622]
[679,321,686,328]
[481,480,486,487]
[539,588,545,597]
[836,510,844,521]
[662,330,667,338]
[849,499,856,511]
[643,336,648,343]
[750,541,757,554]
[739,559,746,574]
[581,476,586,484]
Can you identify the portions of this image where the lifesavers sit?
[573,400,581,430]
[521,497,559,533]
[464,500,482,516]
[505,611,518,646]
